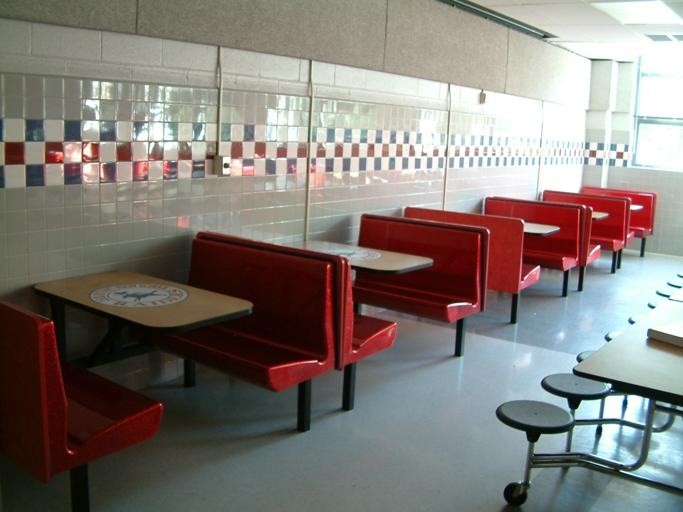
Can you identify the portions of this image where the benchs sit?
[153,228,399,428]
[0,295,169,512]
[485,188,658,299]
[405,202,543,323]
[351,212,492,360]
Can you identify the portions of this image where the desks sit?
[576,298,683,496]
[36,267,255,342]
[275,238,434,277]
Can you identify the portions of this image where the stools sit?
[649,266,683,297]
